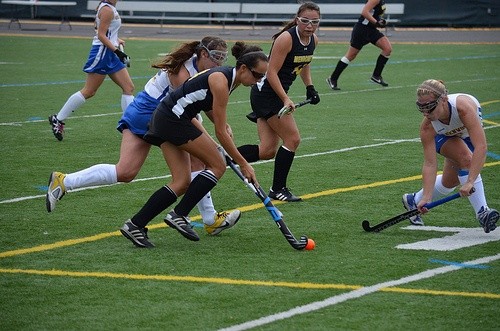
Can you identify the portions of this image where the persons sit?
[326,0,392,90]
[401,78,500,232]
[223,3,320,204]
[48,0,136,142]
[45,35,268,249]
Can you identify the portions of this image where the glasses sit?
[415,92,443,114]
[203,46,229,64]
[239,62,265,79]
[296,17,320,26]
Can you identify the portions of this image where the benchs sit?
[80,0,405,37]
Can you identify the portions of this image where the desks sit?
[1,1,77,32]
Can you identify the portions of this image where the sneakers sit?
[46,171,66,213]
[164,209,200,241]
[476,205,500,233]
[48,113,65,141]
[326,77,341,90]
[120,219,154,247]
[402,192,423,225]
[369,76,388,86]
[268,186,302,203]
[204,210,241,235]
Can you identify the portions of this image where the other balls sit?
[305,238,315,250]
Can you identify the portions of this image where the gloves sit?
[114,49,130,67]
[376,19,386,28]
[305,85,320,105]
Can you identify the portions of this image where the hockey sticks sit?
[277,97,312,119]
[225,155,308,251]
[120,44,130,68]
[362,187,476,233]
